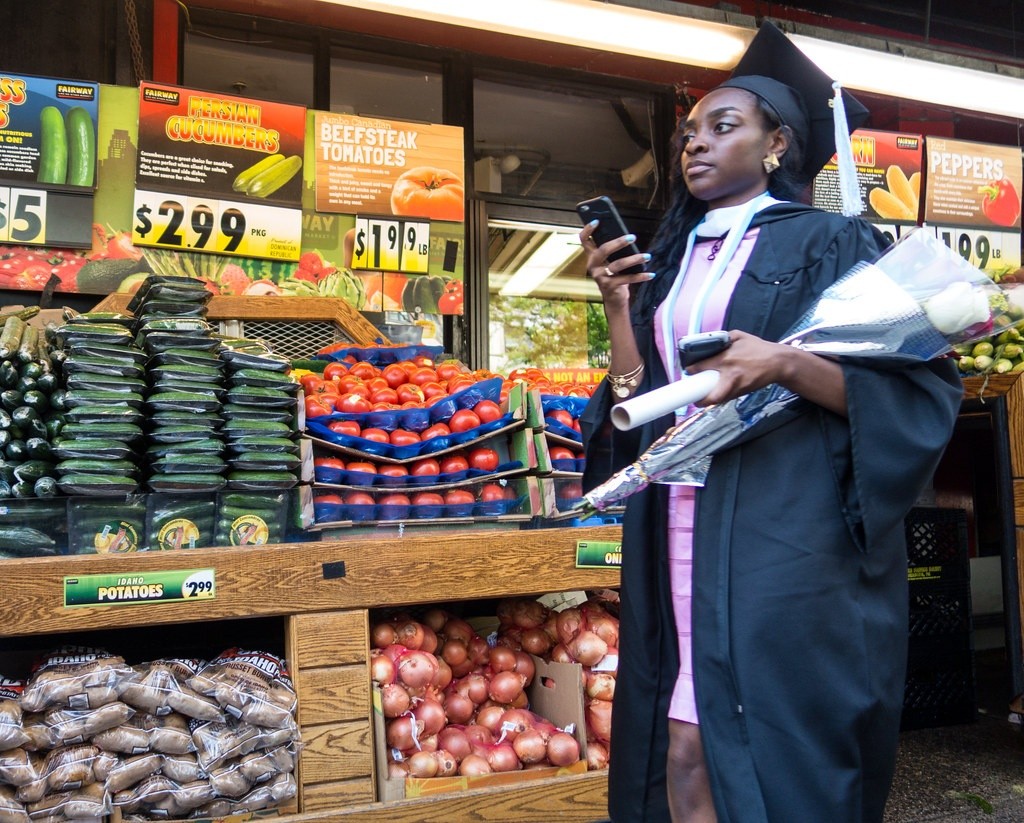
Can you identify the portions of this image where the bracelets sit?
[606,360,645,398]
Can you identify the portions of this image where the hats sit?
[713,17,873,219]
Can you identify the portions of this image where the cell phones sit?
[577,196,647,274]
[678,331,731,370]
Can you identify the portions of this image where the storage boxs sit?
[898,506,973,733]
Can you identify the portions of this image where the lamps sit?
[499,231,587,297]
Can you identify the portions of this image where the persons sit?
[580,75,964,823]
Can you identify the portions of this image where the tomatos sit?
[298,350,603,546]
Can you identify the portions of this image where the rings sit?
[604,266,614,276]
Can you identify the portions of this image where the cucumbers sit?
[0,298,296,550]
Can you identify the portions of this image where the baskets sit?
[898,506,980,732]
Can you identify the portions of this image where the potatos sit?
[0,652,298,823]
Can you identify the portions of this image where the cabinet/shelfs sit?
[0,522,623,822]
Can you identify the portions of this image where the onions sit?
[366,597,620,801]
